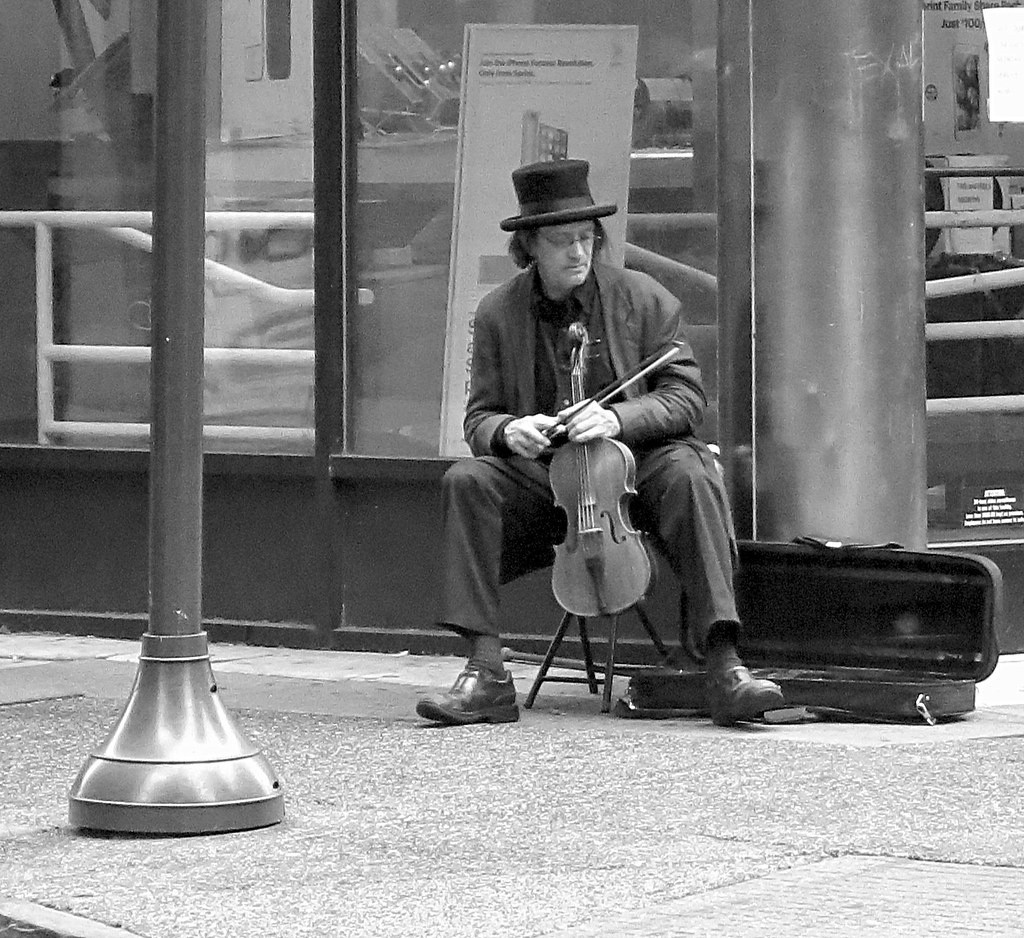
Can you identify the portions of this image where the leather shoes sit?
[706,666,785,726]
[416,670,521,722]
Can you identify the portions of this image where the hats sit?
[499,160,619,231]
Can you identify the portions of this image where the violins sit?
[547,321,661,620]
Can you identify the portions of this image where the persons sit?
[415,159,783,727]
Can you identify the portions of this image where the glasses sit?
[538,223,603,258]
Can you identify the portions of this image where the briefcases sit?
[614,535,1003,728]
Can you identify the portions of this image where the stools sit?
[523,527,671,714]
